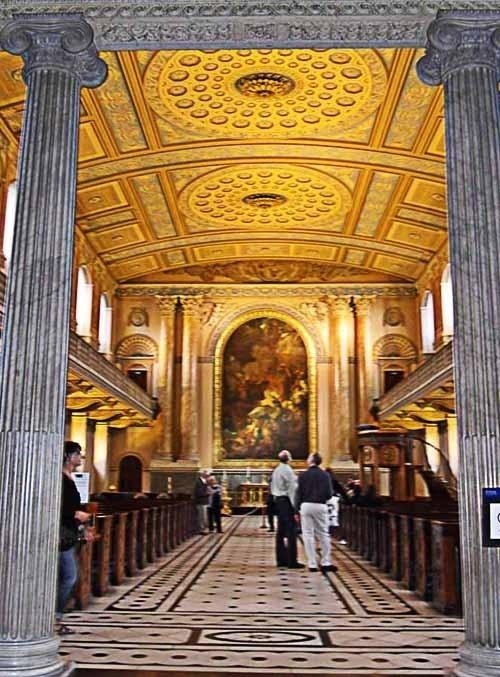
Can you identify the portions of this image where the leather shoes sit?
[277,562,337,572]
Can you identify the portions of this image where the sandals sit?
[56,623,75,634]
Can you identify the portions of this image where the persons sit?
[192,469,212,534]
[268,448,306,569]
[291,450,338,572]
[54,440,101,634]
[265,464,362,533]
[205,474,226,534]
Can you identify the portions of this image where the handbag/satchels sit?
[326,496,339,527]
